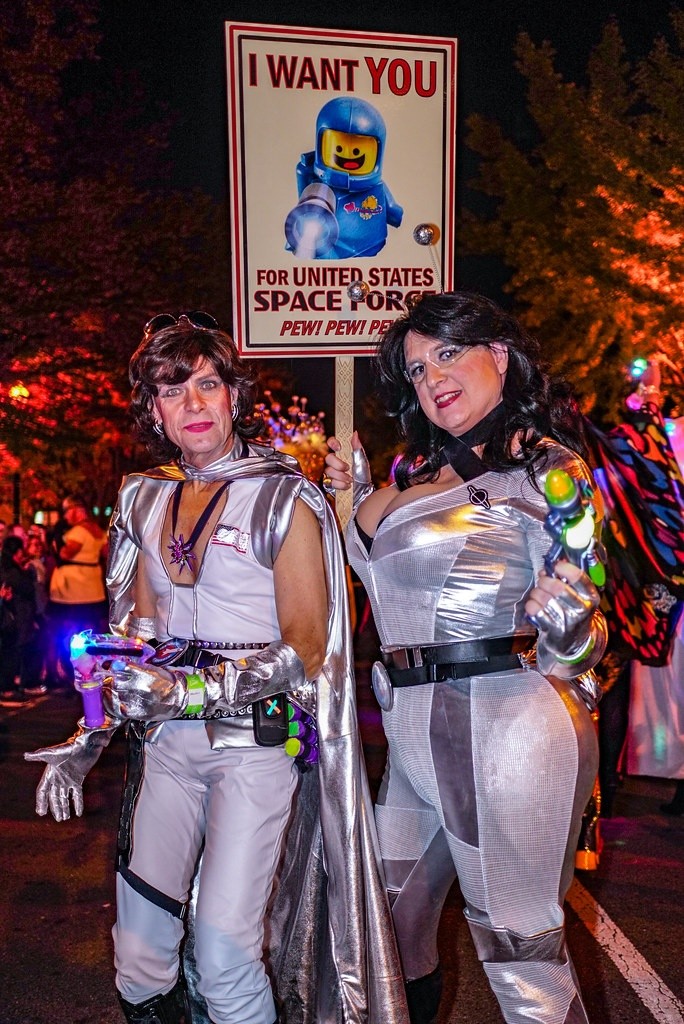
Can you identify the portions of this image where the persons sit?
[24,309,406,1024]
[0,494,109,707]
[324,293,608,1024]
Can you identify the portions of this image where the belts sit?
[382,634,541,688]
[152,642,233,670]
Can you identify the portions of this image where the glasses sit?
[143,311,219,337]
[403,343,474,384]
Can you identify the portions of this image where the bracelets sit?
[557,632,597,664]
[186,674,206,714]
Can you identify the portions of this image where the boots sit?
[116,965,193,1024]
[405,961,443,1024]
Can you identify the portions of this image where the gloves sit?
[101,642,306,721]
[23,712,122,822]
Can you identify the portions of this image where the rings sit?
[323,478,334,487]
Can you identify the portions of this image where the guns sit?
[70,631,156,728]
[530,471,608,630]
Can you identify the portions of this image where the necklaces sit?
[170,435,249,577]
